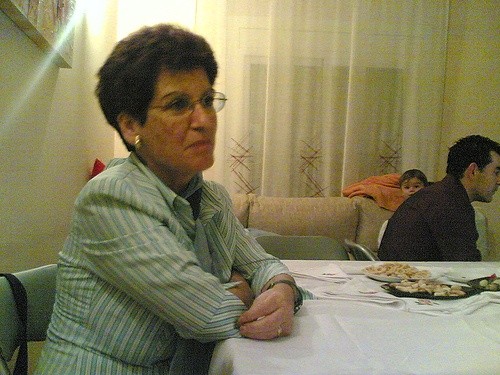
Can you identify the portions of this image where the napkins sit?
[290,263,489,317]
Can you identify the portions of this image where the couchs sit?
[229,190,488,261]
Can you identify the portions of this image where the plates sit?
[362,264,441,283]
[378,275,500,300]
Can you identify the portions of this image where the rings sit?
[277,327,282,337]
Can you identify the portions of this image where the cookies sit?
[374,262,467,297]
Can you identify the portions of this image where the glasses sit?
[152,91,228,115]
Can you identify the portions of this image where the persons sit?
[376,134,500,262]
[378,169,434,249]
[33,23,303,375]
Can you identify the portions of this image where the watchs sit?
[268,279,303,314]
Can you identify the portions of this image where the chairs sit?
[0,234,379,375]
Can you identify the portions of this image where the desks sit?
[206,259,500,375]
